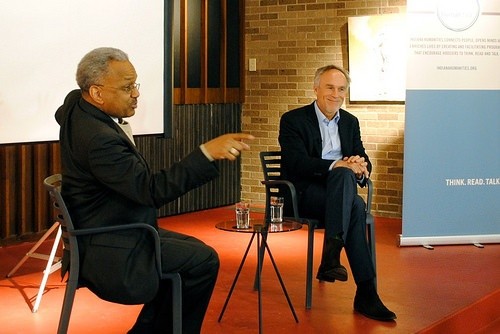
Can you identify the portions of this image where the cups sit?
[235,202,250,228]
[270,196,284,224]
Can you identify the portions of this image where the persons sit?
[278,64,397,320]
[54,46,255,334]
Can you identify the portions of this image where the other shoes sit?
[353,286,396,321]
[315,262,348,283]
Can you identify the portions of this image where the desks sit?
[215,219,302,334]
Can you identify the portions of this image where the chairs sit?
[254,151,376,309]
[44,174,183,334]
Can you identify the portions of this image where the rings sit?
[231,148,236,152]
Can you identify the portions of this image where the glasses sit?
[91,82,140,93]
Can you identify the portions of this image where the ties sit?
[118,117,133,144]
